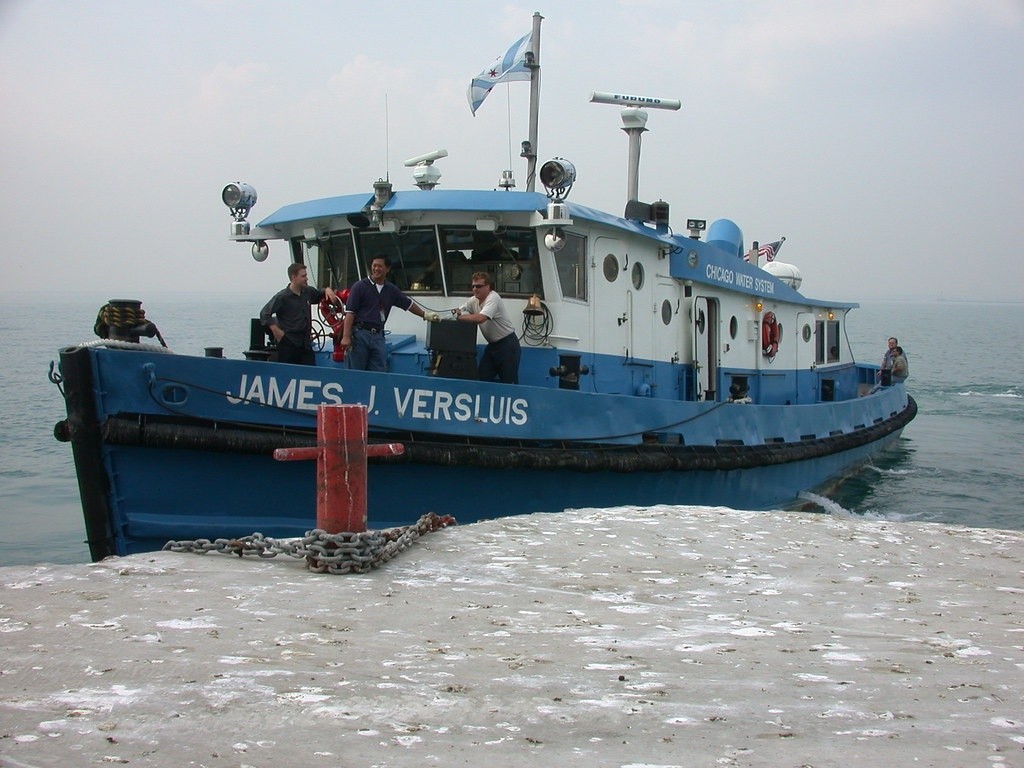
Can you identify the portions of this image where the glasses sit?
[471,285,487,289]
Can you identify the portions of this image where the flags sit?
[742,240,780,262]
[465,29,532,117]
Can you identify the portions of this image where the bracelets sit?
[456,313,461,320]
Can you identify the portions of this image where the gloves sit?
[424,311,440,323]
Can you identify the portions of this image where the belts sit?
[354,323,384,335]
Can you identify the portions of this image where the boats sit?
[47,12,921,566]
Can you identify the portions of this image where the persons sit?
[340,255,439,372]
[451,272,521,385]
[260,263,337,367]
[860,337,909,398]
